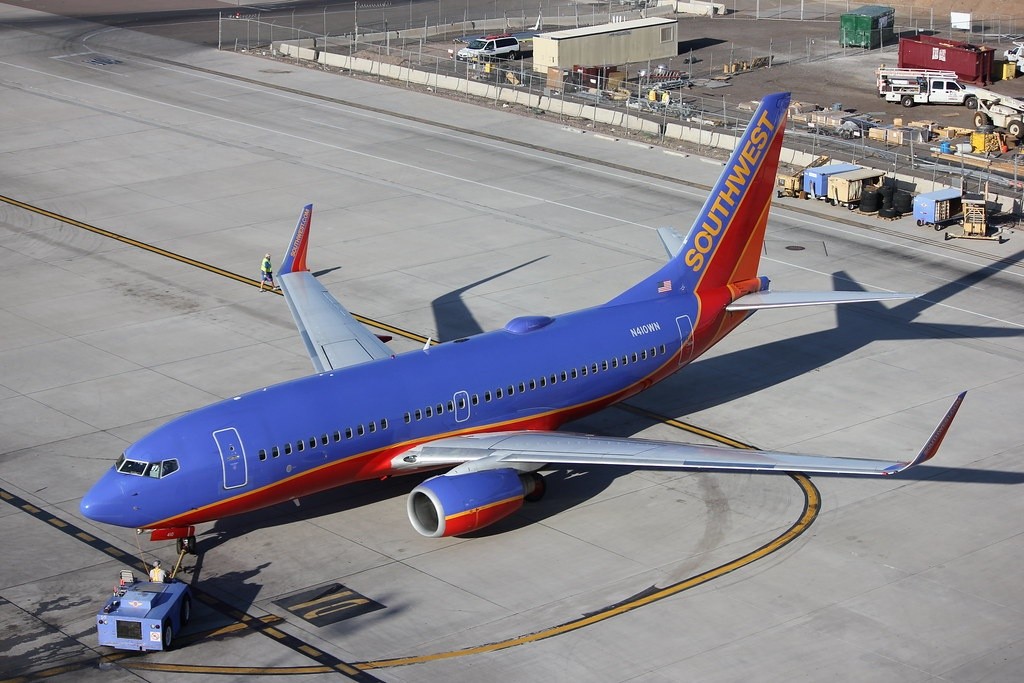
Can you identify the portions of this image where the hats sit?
[152,560,161,568]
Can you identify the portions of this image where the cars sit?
[457,36,521,64]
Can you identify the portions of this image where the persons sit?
[151,463,165,474]
[150,560,168,583]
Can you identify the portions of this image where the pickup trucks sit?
[875,68,979,109]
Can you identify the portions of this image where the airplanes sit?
[81,92,969,554]
